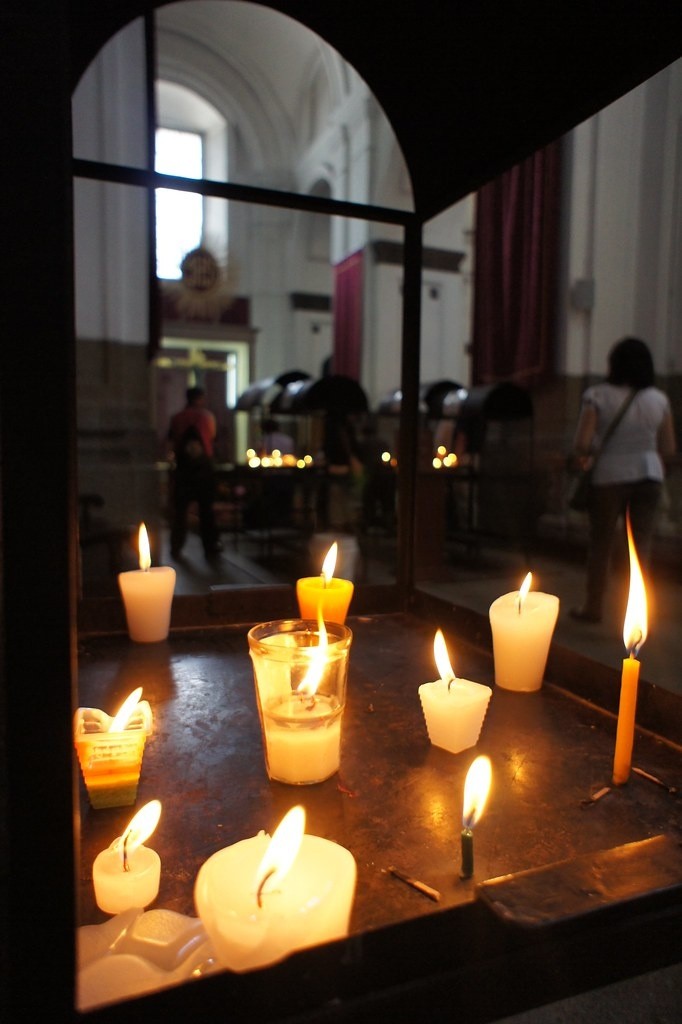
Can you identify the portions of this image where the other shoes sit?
[568,605,602,622]
[170,548,182,559]
[205,544,223,558]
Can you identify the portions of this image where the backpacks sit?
[170,409,207,474]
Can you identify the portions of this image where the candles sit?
[460,756,489,877]
[195,805,356,972]
[613,509,650,784]
[72,685,151,809]
[420,626,492,755]
[298,540,355,626]
[116,523,176,645]
[487,573,562,695]
[249,617,354,784]
[93,799,165,916]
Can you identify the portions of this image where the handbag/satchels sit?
[563,469,593,512]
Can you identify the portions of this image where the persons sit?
[268,354,369,470]
[569,338,674,622]
[160,386,224,561]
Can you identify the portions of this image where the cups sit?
[248,619,352,785]
[74,701,150,809]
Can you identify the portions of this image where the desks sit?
[74,580,682,1024]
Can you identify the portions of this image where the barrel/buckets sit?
[312,529,359,580]
